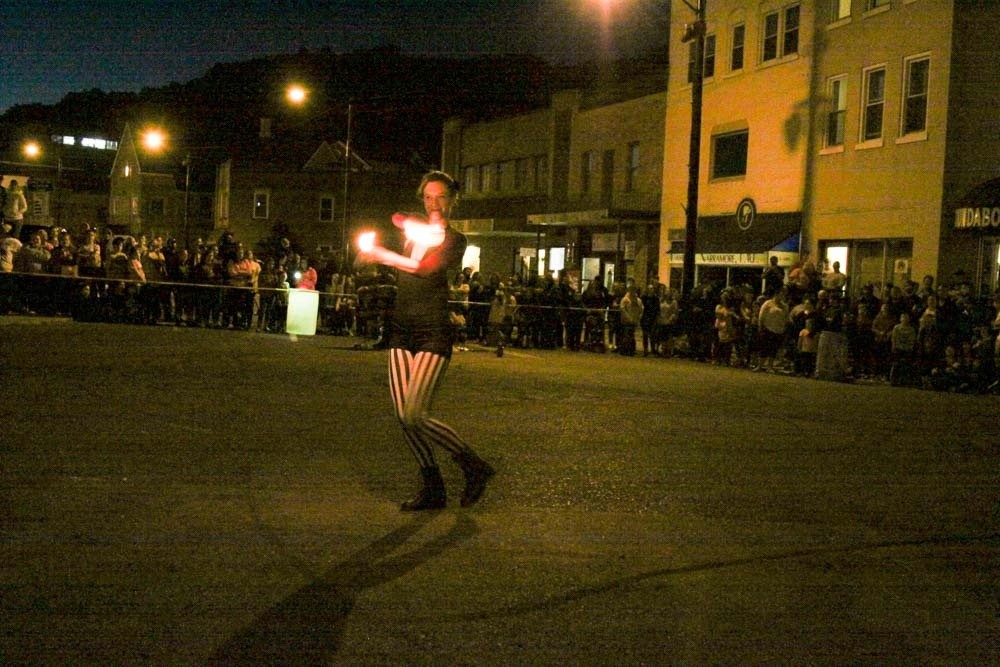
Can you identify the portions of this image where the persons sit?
[447,255,1000,399]
[0,173,404,338]
[359,172,495,513]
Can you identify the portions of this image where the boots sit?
[401,464,448,510]
[765,356,778,374]
[750,356,767,372]
[451,444,496,508]
